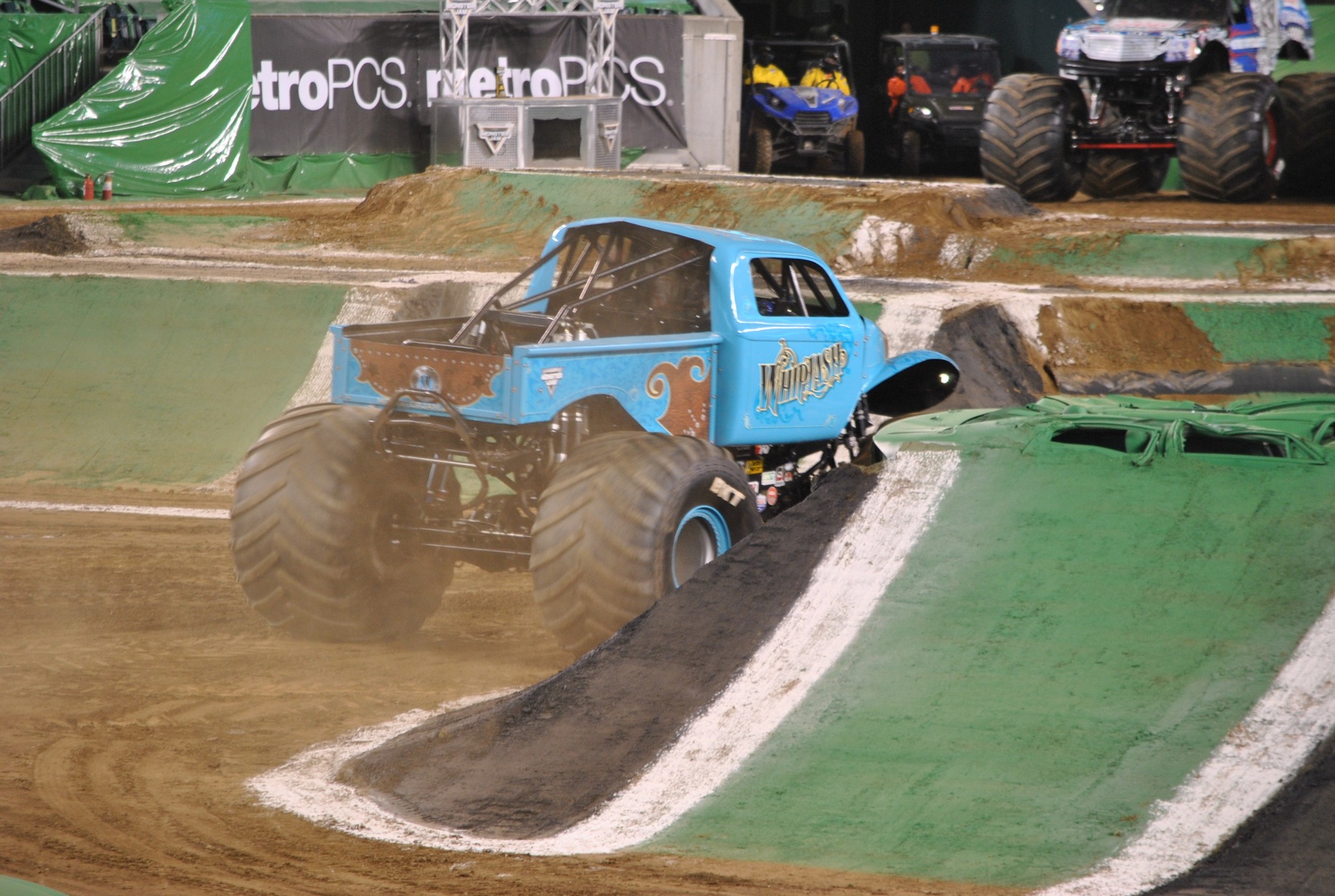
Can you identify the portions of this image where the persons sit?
[951,56,995,94]
[745,45,790,89]
[887,56,933,113]
[799,53,851,97]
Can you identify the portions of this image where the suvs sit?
[977,0,1335,207]
[225,216,963,655]
[867,26,1005,176]
[740,30,867,179]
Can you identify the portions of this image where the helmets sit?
[758,47,774,68]
[820,51,840,73]
[964,56,984,77]
[895,53,913,80]
